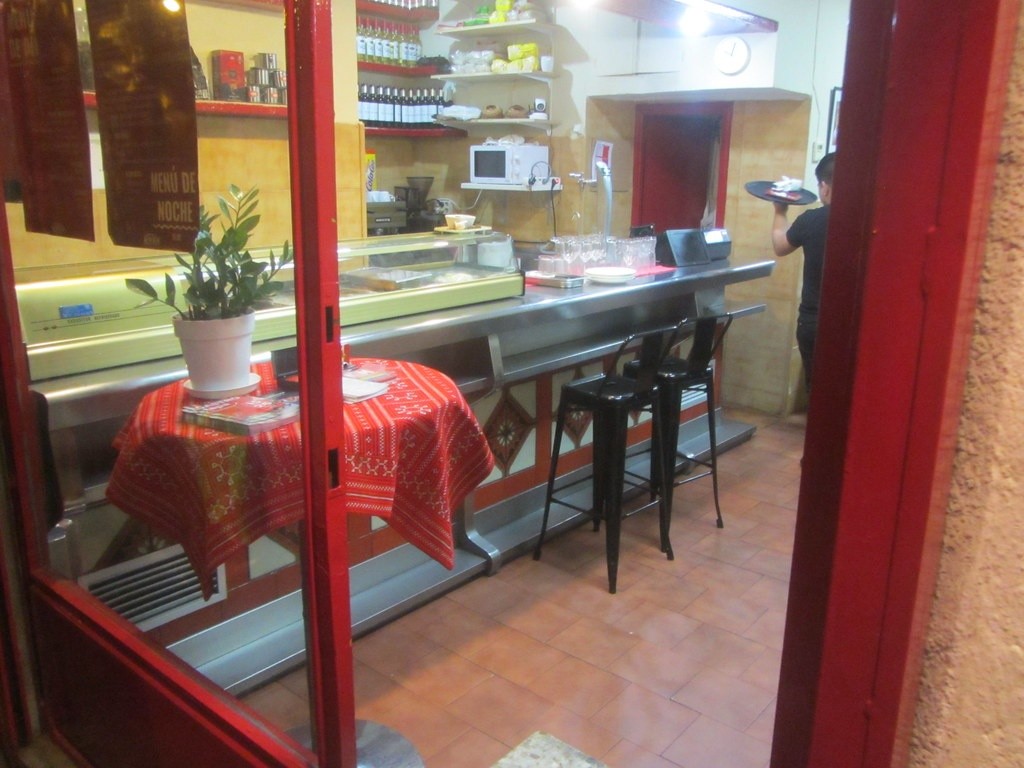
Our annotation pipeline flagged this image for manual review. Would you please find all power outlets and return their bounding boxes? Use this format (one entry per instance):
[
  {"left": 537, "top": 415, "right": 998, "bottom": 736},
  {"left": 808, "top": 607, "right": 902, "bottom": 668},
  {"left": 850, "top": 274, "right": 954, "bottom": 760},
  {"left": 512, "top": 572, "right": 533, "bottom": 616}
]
[{"left": 435, "top": 199, "right": 453, "bottom": 216}]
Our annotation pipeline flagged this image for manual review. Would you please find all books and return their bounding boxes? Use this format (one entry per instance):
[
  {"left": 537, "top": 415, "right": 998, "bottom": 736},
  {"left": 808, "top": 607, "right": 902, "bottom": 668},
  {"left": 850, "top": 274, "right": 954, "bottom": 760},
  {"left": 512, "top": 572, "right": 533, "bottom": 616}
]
[{"left": 183, "top": 393, "right": 300, "bottom": 435}]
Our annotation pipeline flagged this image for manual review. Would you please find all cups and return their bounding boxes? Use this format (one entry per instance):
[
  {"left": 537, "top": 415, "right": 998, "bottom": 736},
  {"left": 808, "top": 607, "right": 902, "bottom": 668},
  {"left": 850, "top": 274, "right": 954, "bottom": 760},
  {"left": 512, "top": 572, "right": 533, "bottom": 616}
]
[
  {"left": 445, "top": 214, "right": 476, "bottom": 229},
  {"left": 539, "top": 234, "right": 658, "bottom": 278},
  {"left": 368, "top": 191, "right": 379, "bottom": 202},
  {"left": 246, "top": 53, "right": 288, "bottom": 105},
  {"left": 379, "top": 191, "right": 395, "bottom": 202}
]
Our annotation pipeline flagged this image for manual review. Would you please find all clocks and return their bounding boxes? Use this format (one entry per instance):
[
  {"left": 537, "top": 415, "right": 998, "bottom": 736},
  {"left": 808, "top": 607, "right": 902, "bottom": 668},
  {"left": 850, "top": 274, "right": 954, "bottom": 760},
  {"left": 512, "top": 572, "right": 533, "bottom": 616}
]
[
  {"left": 713, "top": 36, "right": 751, "bottom": 75},
  {"left": 825, "top": 86, "right": 844, "bottom": 153}
]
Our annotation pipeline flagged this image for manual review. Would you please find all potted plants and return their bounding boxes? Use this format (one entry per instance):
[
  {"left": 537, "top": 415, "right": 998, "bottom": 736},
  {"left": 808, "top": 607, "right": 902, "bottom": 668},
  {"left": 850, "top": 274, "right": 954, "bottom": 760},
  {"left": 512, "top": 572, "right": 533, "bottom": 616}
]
[{"left": 124, "top": 183, "right": 294, "bottom": 397}]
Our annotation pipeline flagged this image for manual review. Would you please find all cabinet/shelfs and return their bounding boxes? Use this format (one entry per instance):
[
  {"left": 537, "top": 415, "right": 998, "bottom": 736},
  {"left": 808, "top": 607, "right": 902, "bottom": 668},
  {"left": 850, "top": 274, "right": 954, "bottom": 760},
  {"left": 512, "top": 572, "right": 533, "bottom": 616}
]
[
  {"left": 357, "top": 0, "right": 467, "bottom": 137},
  {"left": 432, "top": 18, "right": 558, "bottom": 135}
]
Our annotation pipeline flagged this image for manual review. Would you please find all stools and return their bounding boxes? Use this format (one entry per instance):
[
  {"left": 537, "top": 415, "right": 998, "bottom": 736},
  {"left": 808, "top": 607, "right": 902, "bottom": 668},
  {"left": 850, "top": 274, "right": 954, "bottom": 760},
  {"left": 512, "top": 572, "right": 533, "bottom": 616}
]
[
  {"left": 532, "top": 325, "right": 680, "bottom": 594},
  {"left": 622, "top": 313, "right": 735, "bottom": 558}
]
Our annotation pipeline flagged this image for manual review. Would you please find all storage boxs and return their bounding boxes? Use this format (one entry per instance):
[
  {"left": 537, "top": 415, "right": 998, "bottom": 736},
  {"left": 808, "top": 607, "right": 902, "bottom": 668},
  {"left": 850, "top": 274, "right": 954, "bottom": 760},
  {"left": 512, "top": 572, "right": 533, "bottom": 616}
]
[{"left": 212, "top": 50, "right": 246, "bottom": 101}]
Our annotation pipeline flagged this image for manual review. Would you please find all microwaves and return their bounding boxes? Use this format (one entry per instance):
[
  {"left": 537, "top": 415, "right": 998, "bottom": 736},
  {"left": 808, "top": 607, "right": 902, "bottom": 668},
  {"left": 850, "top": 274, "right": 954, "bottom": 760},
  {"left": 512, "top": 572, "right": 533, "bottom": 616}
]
[{"left": 470, "top": 145, "right": 549, "bottom": 184}]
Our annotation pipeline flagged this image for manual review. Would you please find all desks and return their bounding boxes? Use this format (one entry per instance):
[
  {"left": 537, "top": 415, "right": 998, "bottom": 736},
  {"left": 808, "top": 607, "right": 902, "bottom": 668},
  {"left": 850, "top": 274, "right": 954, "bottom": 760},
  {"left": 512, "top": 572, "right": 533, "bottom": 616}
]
[{"left": 104, "top": 358, "right": 493, "bottom": 768}]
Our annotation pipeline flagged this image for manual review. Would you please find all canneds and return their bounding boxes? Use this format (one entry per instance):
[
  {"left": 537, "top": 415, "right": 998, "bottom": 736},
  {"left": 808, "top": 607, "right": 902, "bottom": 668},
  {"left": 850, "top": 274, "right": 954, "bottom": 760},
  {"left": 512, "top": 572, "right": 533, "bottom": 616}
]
[{"left": 248, "top": 53, "right": 287, "bottom": 105}]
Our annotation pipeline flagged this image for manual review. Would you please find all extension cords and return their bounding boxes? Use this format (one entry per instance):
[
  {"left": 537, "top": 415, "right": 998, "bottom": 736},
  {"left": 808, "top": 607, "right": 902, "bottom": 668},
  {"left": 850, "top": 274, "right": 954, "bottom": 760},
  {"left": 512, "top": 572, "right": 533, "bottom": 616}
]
[{"left": 525, "top": 177, "right": 561, "bottom": 186}]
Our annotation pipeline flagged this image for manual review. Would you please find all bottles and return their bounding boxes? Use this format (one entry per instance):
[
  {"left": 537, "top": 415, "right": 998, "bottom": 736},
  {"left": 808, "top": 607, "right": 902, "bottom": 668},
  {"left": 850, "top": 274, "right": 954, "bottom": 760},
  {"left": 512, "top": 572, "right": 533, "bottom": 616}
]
[
  {"left": 358, "top": 84, "right": 453, "bottom": 128},
  {"left": 356, "top": 15, "right": 422, "bottom": 68},
  {"left": 364, "top": 0, "right": 438, "bottom": 11}
]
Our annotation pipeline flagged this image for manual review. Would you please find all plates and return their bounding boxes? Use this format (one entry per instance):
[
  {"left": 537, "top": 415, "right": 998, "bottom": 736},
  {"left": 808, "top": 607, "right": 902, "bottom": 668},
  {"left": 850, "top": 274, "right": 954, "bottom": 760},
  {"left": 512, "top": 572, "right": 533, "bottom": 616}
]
[
  {"left": 743, "top": 180, "right": 818, "bottom": 205},
  {"left": 434, "top": 226, "right": 492, "bottom": 233},
  {"left": 584, "top": 267, "right": 635, "bottom": 283}
]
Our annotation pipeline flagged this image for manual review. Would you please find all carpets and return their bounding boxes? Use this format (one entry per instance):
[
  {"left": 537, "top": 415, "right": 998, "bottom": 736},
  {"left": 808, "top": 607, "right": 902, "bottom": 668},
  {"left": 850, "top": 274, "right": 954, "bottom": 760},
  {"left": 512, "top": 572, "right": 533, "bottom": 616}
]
[{"left": 493, "top": 728, "right": 606, "bottom": 768}]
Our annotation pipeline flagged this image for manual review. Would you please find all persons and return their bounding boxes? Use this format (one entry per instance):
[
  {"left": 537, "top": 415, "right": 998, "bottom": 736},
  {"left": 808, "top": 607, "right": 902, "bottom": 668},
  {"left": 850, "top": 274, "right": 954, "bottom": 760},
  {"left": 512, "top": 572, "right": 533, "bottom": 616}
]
[{"left": 772, "top": 153, "right": 837, "bottom": 468}]
